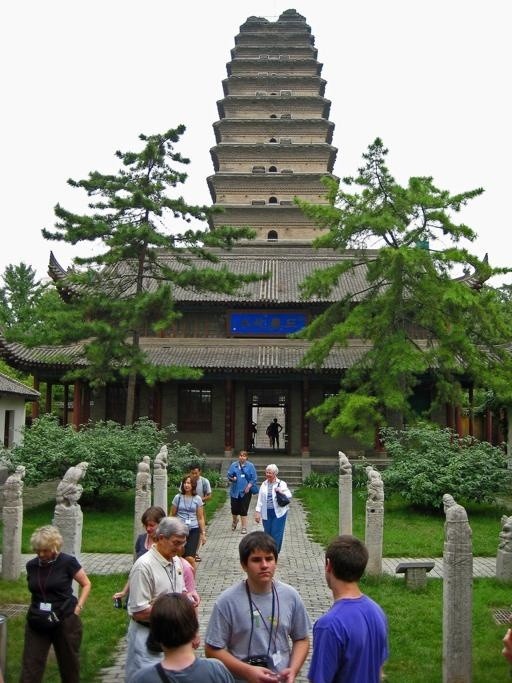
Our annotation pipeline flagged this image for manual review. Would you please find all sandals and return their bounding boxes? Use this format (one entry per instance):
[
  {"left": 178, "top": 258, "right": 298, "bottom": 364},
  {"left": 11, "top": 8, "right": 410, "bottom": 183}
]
[
  {"left": 194, "top": 554, "right": 200, "bottom": 562},
  {"left": 232, "top": 519, "right": 239, "bottom": 532},
  {"left": 241, "top": 528, "right": 247, "bottom": 534}
]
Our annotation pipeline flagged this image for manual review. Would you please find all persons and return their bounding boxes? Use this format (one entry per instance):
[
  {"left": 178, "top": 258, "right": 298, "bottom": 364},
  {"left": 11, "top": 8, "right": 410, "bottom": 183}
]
[
  {"left": 270, "top": 418, "right": 283, "bottom": 449},
  {"left": 266, "top": 422, "right": 273, "bottom": 447},
  {"left": 308, "top": 533, "right": 390, "bottom": 683},
  {"left": 187, "top": 462, "right": 213, "bottom": 505},
  {"left": 172, "top": 476, "right": 207, "bottom": 568},
  {"left": 123, "top": 517, "right": 202, "bottom": 682},
  {"left": 172, "top": 551, "right": 197, "bottom": 611},
  {"left": 111, "top": 506, "right": 167, "bottom": 610},
  {"left": 252, "top": 421, "right": 257, "bottom": 448},
  {"left": 206, "top": 531, "right": 311, "bottom": 683},
  {"left": 124, "top": 591, "right": 234, "bottom": 683},
  {"left": 255, "top": 464, "right": 292, "bottom": 556},
  {"left": 227, "top": 448, "right": 258, "bottom": 535},
  {"left": 19, "top": 523, "right": 91, "bottom": 683}
]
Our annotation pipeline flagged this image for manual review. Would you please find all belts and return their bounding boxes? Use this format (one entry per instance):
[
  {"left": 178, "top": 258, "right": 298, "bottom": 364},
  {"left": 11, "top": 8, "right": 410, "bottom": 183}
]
[{"left": 131, "top": 616, "right": 149, "bottom": 629}]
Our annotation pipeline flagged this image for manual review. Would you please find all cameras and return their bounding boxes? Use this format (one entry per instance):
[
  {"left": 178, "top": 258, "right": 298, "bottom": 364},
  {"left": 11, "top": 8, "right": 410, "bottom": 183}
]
[{"left": 113, "top": 599, "right": 122, "bottom": 608}]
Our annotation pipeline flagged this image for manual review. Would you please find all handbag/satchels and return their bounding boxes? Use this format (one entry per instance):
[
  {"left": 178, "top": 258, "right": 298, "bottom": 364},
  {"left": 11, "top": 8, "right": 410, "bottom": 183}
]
[
  {"left": 276, "top": 491, "right": 291, "bottom": 506},
  {"left": 250, "top": 486, "right": 259, "bottom": 495},
  {"left": 25, "top": 609, "right": 65, "bottom": 635}
]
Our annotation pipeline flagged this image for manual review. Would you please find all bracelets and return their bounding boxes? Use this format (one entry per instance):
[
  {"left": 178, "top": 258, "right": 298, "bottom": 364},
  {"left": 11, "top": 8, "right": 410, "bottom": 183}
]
[
  {"left": 200, "top": 531, "right": 206, "bottom": 534},
  {"left": 77, "top": 603, "right": 82, "bottom": 609}
]
